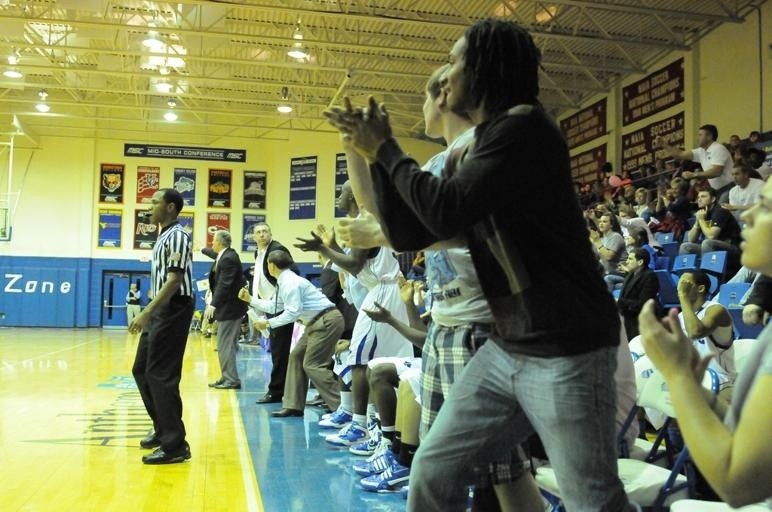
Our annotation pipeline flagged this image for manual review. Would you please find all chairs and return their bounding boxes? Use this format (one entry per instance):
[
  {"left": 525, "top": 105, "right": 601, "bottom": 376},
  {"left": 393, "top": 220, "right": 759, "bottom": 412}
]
[
  {"left": 644, "top": 231, "right": 764, "bottom": 340},
  {"left": 535, "top": 367, "right": 719, "bottom": 510}
]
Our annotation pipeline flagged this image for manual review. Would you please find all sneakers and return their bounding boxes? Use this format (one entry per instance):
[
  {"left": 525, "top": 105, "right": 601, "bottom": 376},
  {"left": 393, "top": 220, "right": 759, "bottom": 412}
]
[
  {"left": 208, "top": 380, "right": 223, "bottom": 387},
  {"left": 142, "top": 442, "right": 191, "bottom": 464},
  {"left": 316, "top": 405, "right": 408, "bottom": 501},
  {"left": 214, "top": 381, "right": 241, "bottom": 390},
  {"left": 202, "top": 330, "right": 212, "bottom": 339},
  {"left": 239, "top": 335, "right": 261, "bottom": 346},
  {"left": 139, "top": 432, "right": 161, "bottom": 450}
]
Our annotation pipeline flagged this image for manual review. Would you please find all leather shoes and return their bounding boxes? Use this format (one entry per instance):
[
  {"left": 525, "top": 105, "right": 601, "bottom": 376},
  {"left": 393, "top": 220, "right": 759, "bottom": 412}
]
[
  {"left": 271, "top": 408, "right": 304, "bottom": 418},
  {"left": 255, "top": 392, "right": 282, "bottom": 404},
  {"left": 306, "top": 398, "right": 325, "bottom": 407}
]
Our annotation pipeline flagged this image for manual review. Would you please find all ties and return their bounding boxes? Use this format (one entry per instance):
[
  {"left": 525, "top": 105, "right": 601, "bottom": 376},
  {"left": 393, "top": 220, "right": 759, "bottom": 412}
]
[{"left": 252, "top": 252, "right": 262, "bottom": 300}]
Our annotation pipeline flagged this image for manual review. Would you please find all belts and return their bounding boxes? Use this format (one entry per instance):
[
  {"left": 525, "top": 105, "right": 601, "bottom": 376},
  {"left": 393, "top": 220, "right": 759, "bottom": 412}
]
[{"left": 307, "top": 305, "right": 337, "bottom": 324}]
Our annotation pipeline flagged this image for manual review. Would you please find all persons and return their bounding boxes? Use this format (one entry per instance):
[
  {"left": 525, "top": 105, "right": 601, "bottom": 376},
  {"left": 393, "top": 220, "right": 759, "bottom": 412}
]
[
  {"left": 200, "top": 265, "right": 272, "bottom": 352},
  {"left": 128, "top": 188, "right": 194, "bottom": 465},
  {"left": 236, "top": 249, "right": 345, "bottom": 417},
  {"left": 252, "top": 222, "right": 300, "bottom": 404},
  {"left": 192, "top": 230, "right": 248, "bottom": 390},
  {"left": 125, "top": 283, "right": 142, "bottom": 334},
  {"left": 295, "top": 19, "right": 771, "bottom": 512}
]
